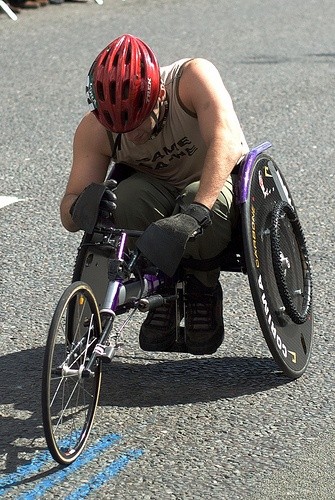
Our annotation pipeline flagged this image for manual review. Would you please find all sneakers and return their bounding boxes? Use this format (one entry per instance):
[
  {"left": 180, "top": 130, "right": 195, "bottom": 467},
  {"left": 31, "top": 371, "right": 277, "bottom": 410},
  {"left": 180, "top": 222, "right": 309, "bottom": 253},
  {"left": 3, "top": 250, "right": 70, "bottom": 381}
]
[{"left": 138, "top": 280, "right": 224, "bottom": 355}]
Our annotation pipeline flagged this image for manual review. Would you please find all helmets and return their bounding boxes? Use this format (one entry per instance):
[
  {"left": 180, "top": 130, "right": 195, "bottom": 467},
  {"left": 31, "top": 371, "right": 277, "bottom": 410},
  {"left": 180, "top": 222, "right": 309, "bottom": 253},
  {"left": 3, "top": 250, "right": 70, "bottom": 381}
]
[{"left": 85, "top": 33, "right": 160, "bottom": 133}]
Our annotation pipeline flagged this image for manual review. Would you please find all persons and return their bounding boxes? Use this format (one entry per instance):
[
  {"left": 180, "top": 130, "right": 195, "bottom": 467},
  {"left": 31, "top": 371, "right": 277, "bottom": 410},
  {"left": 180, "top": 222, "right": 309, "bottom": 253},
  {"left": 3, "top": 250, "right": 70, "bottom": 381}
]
[{"left": 60, "top": 33, "right": 249, "bottom": 356}]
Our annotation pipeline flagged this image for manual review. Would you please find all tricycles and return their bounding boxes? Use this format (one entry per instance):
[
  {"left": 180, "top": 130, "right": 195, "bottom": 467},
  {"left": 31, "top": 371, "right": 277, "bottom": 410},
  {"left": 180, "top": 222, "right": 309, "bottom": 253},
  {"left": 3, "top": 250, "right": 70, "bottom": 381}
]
[{"left": 40, "top": 142, "right": 315, "bottom": 465}]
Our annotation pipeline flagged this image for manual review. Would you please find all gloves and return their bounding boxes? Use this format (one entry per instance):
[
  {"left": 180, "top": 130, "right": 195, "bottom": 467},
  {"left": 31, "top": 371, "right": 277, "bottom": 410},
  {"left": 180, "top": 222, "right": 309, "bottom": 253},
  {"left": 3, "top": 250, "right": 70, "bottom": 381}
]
[
  {"left": 135, "top": 204, "right": 212, "bottom": 278},
  {"left": 69, "top": 178, "right": 117, "bottom": 231}
]
